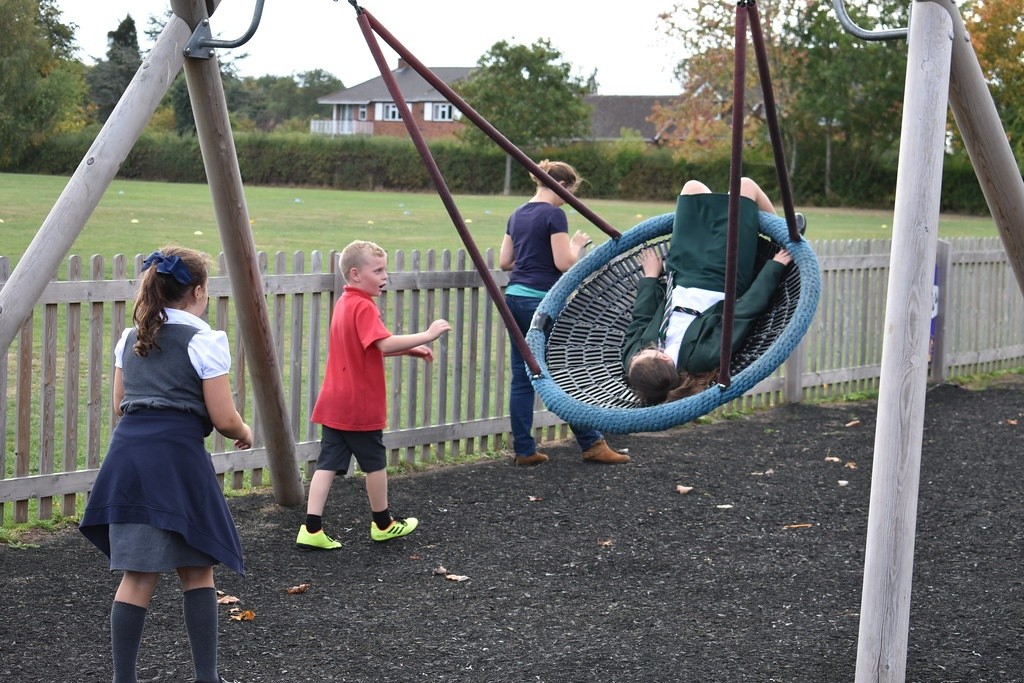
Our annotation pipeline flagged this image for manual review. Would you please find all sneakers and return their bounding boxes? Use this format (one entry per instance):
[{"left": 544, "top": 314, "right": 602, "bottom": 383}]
[
  {"left": 296, "top": 525, "right": 342, "bottom": 549},
  {"left": 371, "top": 515, "right": 418, "bottom": 542}
]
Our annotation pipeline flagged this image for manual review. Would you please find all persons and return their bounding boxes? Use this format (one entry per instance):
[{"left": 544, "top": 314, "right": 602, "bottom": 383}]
[
  {"left": 499, "top": 159, "right": 630, "bottom": 467},
  {"left": 624, "top": 176, "right": 807, "bottom": 404},
  {"left": 295, "top": 241, "right": 451, "bottom": 550},
  {"left": 84, "top": 247, "right": 251, "bottom": 683}
]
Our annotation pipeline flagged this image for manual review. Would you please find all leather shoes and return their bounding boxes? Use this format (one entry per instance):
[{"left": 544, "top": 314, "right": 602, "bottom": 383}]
[{"left": 795, "top": 213, "right": 806, "bottom": 236}]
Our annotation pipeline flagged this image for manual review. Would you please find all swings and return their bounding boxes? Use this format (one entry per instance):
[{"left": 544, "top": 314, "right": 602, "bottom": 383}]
[{"left": 350, "top": 0, "right": 819, "bottom": 432}]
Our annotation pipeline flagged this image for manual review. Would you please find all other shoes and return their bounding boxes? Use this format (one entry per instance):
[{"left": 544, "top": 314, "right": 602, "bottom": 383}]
[
  {"left": 583, "top": 439, "right": 630, "bottom": 463},
  {"left": 516, "top": 451, "right": 548, "bottom": 466}
]
